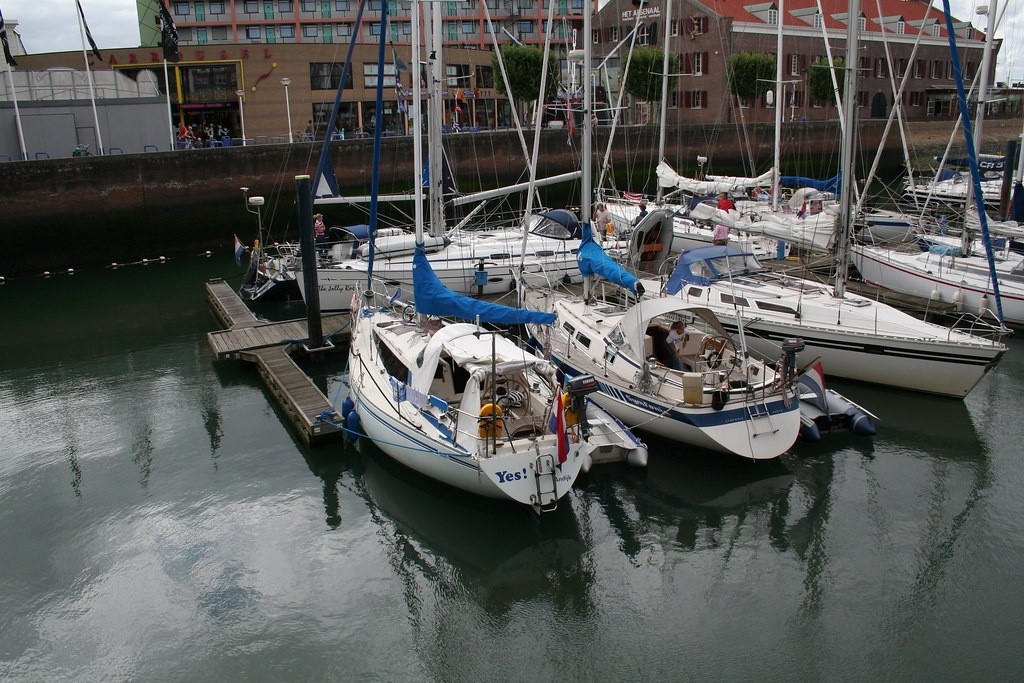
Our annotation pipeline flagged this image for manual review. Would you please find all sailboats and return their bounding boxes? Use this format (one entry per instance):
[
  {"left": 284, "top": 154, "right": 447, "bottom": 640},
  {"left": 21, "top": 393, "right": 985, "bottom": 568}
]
[{"left": 237, "top": 0, "right": 1024, "bottom": 515}]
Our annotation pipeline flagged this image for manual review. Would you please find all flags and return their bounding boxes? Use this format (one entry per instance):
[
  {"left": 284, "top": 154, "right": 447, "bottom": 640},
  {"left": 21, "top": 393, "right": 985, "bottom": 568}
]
[
  {"left": 157, "top": 0, "right": 182, "bottom": 64},
  {"left": 0, "top": 10, "right": 18, "bottom": 68},
  {"left": 568, "top": 103, "right": 575, "bottom": 140},
  {"left": 397, "top": 95, "right": 409, "bottom": 115},
  {"left": 546, "top": 387, "right": 571, "bottom": 464},
  {"left": 395, "top": 74, "right": 409, "bottom": 97},
  {"left": 393, "top": 50, "right": 409, "bottom": 72},
  {"left": 76, "top": 0, "right": 104, "bottom": 62},
  {"left": 456, "top": 88, "right": 469, "bottom": 105}
]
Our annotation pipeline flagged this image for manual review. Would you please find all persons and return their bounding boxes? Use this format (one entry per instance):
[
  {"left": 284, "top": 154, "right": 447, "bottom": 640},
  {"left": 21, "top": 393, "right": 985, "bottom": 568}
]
[
  {"left": 178, "top": 122, "right": 187, "bottom": 141},
  {"left": 665, "top": 321, "right": 688, "bottom": 371},
  {"left": 185, "top": 126, "right": 196, "bottom": 148},
  {"left": 592, "top": 114, "right": 598, "bottom": 127},
  {"left": 716, "top": 191, "right": 737, "bottom": 213},
  {"left": 632, "top": 205, "right": 648, "bottom": 227},
  {"left": 592, "top": 204, "right": 611, "bottom": 241},
  {"left": 313, "top": 214, "right": 325, "bottom": 255},
  {"left": 197, "top": 137, "right": 203, "bottom": 148},
  {"left": 307, "top": 119, "right": 315, "bottom": 141}
]
